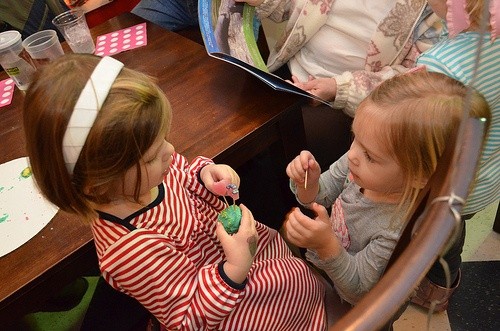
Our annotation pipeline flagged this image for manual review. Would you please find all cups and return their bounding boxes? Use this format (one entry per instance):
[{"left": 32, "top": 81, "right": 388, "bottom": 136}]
[
  {"left": 52, "top": 9, "right": 95, "bottom": 55},
  {"left": 22, "top": 30, "right": 65, "bottom": 66},
  {"left": 0, "top": 31, "right": 37, "bottom": 91}
]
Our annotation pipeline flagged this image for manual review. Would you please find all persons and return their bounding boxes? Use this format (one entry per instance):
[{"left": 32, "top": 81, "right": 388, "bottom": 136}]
[
  {"left": 233, "top": 0, "right": 444, "bottom": 119},
  {"left": 130, "top": 0, "right": 198, "bottom": 37},
  {"left": 412, "top": 0, "right": 500, "bottom": 314},
  {"left": 284, "top": 71, "right": 492, "bottom": 331},
  {"left": 23, "top": 53, "right": 328, "bottom": 331}
]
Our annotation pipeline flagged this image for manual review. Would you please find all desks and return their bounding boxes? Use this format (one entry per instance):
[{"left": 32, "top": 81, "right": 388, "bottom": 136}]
[{"left": 0, "top": 12, "right": 307, "bottom": 331}]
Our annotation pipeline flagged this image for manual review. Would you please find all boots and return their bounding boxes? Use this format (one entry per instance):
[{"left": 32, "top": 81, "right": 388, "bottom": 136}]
[{"left": 411, "top": 269, "right": 461, "bottom": 313}]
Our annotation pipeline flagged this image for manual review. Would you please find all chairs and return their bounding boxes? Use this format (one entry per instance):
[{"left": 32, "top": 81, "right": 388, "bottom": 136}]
[{"left": 328, "top": 117, "right": 484, "bottom": 331}]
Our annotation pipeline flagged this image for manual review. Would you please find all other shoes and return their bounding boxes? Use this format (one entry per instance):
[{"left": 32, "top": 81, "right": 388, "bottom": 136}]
[{"left": 26, "top": 275, "right": 89, "bottom": 312}]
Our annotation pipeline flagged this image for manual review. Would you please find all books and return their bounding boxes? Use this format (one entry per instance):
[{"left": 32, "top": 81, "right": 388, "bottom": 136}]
[{"left": 197, "top": 0, "right": 335, "bottom": 111}]
